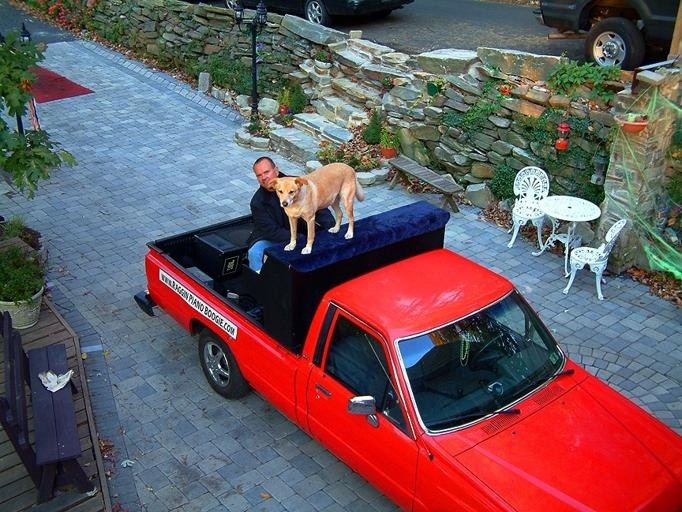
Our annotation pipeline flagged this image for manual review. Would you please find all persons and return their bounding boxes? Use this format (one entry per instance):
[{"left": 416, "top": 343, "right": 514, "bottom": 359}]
[{"left": 244, "top": 157, "right": 336, "bottom": 321}]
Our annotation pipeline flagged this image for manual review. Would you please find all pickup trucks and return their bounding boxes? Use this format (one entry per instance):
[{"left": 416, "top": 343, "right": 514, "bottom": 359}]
[{"left": 135, "top": 209, "right": 682, "bottom": 512}]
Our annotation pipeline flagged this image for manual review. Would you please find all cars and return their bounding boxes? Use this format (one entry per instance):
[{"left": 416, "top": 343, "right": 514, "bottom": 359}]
[
  {"left": 533, "top": 1, "right": 679, "bottom": 68},
  {"left": 225, "top": 0, "right": 416, "bottom": 26}
]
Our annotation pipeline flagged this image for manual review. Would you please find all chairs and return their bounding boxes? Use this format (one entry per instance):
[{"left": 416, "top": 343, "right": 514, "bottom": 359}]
[
  {"left": 506, "top": 166, "right": 550, "bottom": 250},
  {"left": 563, "top": 218, "right": 628, "bottom": 301},
  {"left": 328, "top": 317, "right": 513, "bottom": 422}
]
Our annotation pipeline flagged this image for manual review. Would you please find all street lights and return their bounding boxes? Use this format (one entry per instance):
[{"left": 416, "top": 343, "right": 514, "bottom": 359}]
[
  {"left": 0, "top": 23, "right": 31, "bottom": 139},
  {"left": 233, "top": 0, "right": 268, "bottom": 121}
]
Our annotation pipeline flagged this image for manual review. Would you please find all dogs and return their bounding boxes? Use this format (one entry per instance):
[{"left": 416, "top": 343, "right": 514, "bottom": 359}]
[{"left": 266, "top": 162, "right": 365, "bottom": 255}]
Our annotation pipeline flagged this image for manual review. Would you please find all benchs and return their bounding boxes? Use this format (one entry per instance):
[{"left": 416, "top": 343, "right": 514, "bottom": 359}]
[
  {"left": 388, "top": 152, "right": 467, "bottom": 208},
  {"left": 0, "top": 311, "right": 94, "bottom": 505}
]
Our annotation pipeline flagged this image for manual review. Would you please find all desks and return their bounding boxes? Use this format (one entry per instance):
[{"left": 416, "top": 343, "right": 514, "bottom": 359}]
[{"left": 531, "top": 196, "right": 602, "bottom": 277}]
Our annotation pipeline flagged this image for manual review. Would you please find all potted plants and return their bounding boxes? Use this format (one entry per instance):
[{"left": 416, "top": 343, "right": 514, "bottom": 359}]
[
  {"left": 314, "top": 50, "right": 334, "bottom": 69},
  {"left": 0, "top": 244, "right": 45, "bottom": 329},
  {"left": 380, "top": 132, "right": 400, "bottom": 158}
]
